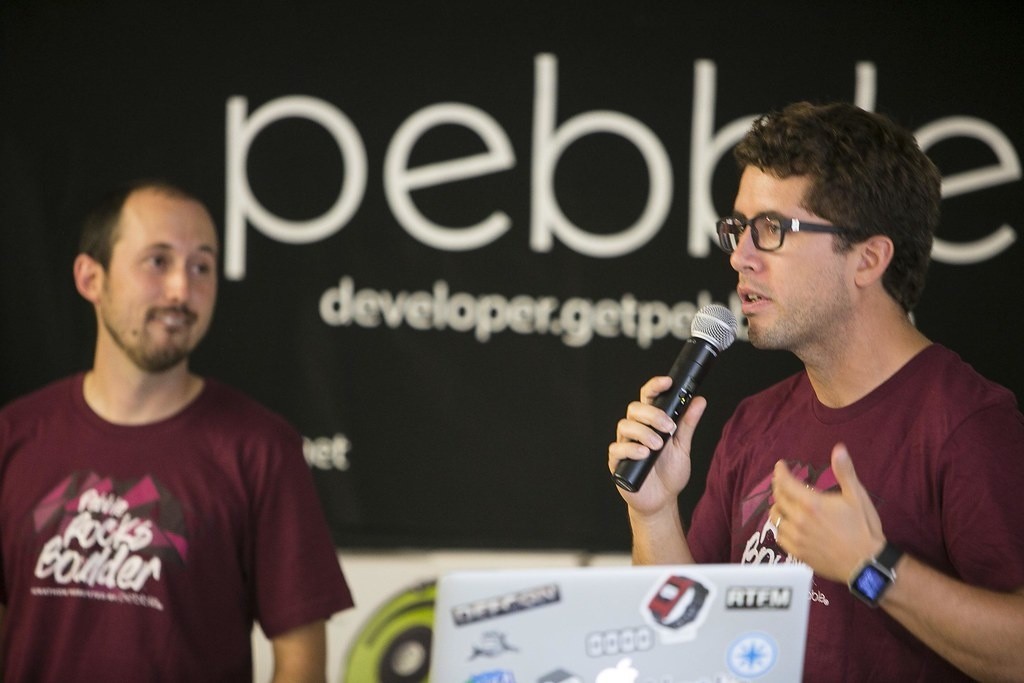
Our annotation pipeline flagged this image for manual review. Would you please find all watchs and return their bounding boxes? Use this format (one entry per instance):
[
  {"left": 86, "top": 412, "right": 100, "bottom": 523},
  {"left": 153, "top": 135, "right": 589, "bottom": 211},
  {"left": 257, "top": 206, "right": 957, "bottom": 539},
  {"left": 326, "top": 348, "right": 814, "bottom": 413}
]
[{"left": 846, "top": 541, "right": 906, "bottom": 609}]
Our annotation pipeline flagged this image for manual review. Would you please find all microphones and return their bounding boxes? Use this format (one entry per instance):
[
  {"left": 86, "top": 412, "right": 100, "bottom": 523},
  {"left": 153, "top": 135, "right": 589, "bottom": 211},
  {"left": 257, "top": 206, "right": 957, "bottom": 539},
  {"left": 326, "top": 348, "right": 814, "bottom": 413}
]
[{"left": 611, "top": 304, "right": 736, "bottom": 493}]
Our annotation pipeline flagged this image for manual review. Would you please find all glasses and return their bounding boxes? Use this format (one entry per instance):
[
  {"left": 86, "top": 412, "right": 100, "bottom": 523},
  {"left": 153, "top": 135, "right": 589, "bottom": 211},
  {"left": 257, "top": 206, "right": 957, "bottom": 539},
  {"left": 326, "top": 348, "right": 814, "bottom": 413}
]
[{"left": 715, "top": 214, "right": 873, "bottom": 254}]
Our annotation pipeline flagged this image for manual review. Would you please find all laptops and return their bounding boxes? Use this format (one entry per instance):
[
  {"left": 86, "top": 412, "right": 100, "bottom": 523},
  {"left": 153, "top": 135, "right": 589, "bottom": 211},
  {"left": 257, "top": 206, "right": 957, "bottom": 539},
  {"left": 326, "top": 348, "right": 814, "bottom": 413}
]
[{"left": 426, "top": 563, "right": 814, "bottom": 683}]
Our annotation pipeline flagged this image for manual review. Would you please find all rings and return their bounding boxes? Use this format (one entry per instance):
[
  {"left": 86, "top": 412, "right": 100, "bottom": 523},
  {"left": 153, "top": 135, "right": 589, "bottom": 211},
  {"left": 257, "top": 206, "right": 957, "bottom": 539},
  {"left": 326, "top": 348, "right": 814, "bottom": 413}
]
[{"left": 775, "top": 517, "right": 783, "bottom": 530}]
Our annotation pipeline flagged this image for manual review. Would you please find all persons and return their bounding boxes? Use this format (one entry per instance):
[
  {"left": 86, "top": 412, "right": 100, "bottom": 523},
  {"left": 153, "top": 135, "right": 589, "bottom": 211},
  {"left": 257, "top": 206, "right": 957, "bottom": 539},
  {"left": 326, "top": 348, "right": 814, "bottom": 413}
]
[
  {"left": 0, "top": 169, "right": 356, "bottom": 683},
  {"left": 606, "top": 102, "right": 1023, "bottom": 683}
]
